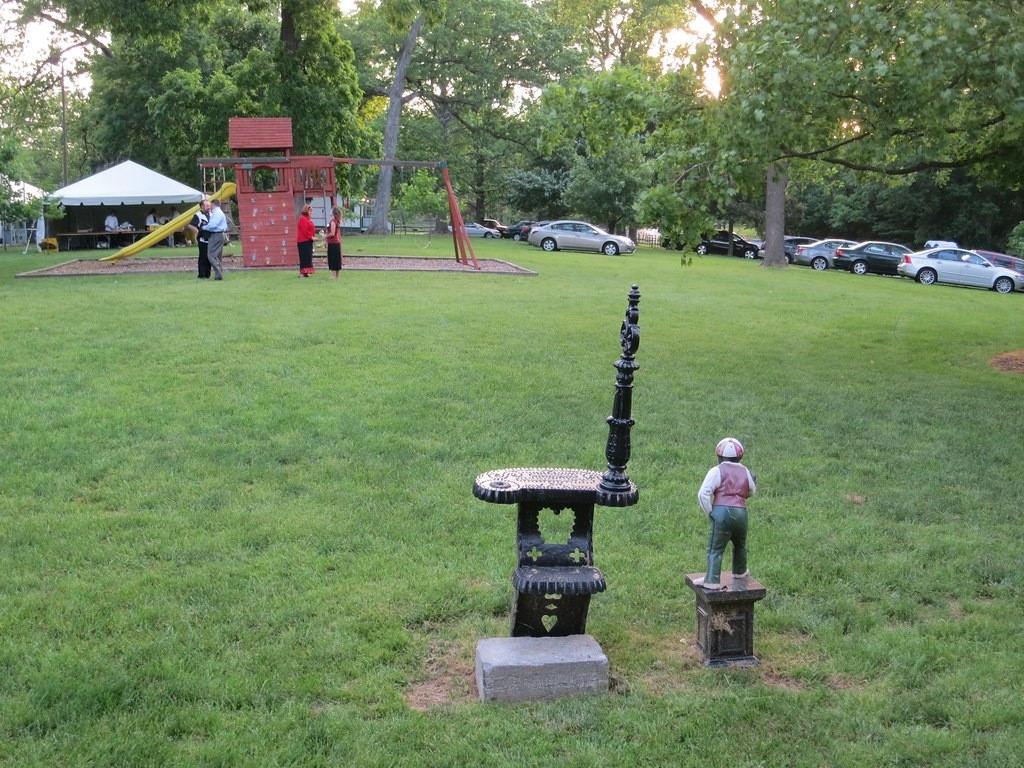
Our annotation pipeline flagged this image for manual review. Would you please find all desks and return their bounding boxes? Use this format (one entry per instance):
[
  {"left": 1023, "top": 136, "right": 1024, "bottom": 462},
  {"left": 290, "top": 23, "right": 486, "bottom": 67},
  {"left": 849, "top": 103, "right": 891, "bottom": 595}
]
[{"left": 56, "top": 230, "right": 184, "bottom": 251}]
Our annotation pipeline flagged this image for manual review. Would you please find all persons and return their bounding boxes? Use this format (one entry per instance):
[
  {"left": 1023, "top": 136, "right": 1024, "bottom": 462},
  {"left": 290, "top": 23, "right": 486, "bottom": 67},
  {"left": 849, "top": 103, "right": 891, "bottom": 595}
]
[
  {"left": 146, "top": 207, "right": 188, "bottom": 247},
  {"left": 297, "top": 205, "right": 319, "bottom": 277},
  {"left": 105, "top": 210, "right": 118, "bottom": 249},
  {"left": 189, "top": 198, "right": 227, "bottom": 280},
  {"left": 322, "top": 206, "right": 343, "bottom": 280},
  {"left": 692, "top": 438, "right": 756, "bottom": 589}
]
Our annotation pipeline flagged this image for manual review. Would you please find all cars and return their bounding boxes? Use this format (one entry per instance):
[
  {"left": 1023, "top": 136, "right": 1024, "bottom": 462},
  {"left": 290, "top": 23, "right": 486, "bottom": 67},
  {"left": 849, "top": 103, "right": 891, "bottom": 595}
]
[
  {"left": 683, "top": 229, "right": 759, "bottom": 259},
  {"left": 923, "top": 240, "right": 1024, "bottom": 274},
  {"left": 526, "top": 219, "right": 636, "bottom": 257},
  {"left": 831, "top": 239, "right": 916, "bottom": 277},
  {"left": 896, "top": 247, "right": 1024, "bottom": 294},
  {"left": 662, "top": 232, "right": 692, "bottom": 251},
  {"left": 447, "top": 216, "right": 595, "bottom": 242},
  {"left": 759, "top": 234, "right": 833, "bottom": 266},
  {"left": 793, "top": 239, "right": 895, "bottom": 272}
]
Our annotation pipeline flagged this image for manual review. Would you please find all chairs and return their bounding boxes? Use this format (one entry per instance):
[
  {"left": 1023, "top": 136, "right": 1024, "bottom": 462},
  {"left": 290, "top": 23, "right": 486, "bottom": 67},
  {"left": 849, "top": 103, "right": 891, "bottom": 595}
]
[
  {"left": 575, "top": 226, "right": 582, "bottom": 232},
  {"left": 882, "top": 249, "right": 889, "bottom": 255}
]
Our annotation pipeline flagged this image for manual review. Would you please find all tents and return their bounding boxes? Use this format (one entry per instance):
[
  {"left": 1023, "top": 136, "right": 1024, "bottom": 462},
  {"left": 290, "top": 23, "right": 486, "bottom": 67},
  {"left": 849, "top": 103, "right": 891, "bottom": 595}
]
[
  {"left": 37, "top": 160, "right": 211, "bottom": 252},
  {"left": 0, "top": 173, "right": 50, "bottom": 243}
]
